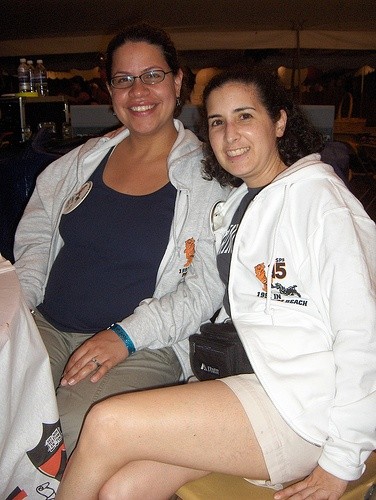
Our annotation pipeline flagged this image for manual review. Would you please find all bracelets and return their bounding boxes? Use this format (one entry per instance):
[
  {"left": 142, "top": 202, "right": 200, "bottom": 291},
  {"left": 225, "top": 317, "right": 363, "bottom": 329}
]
[{"left": 110, "top": 324, "right": 135, "bottom": 356}]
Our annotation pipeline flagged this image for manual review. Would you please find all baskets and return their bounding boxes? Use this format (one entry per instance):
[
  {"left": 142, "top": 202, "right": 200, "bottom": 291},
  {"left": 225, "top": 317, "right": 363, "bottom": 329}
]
[{"left": 334, "top": 93, "right": 366, "bottom": 140}]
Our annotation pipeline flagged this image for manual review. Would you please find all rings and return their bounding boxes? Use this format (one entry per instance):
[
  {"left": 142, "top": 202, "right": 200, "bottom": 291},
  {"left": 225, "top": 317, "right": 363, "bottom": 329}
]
[{"left": 91, "top": 360, "right": 100, "bottom": 367}]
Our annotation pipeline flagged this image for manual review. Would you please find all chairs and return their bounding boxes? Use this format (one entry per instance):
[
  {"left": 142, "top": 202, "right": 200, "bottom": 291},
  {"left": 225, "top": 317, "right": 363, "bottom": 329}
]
[
  {"left": 335, "top": 139, "right": 376, "bottom": 211},
  {"left": 173, "top": 449, "right": 376, "bottom": 500}
]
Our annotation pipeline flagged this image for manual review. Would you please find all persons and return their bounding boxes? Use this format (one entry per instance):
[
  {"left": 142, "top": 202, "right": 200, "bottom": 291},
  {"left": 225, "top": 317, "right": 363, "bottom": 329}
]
[
  {"left": 55, "top": 60, "right": 376, "bottom": 500},
  {"left": 14, "top": 24, "right": 233, "bottom": 461},
  {"left": 299, "top": 67, "right": 325, "bottom": 106},
  {"left": 66, "top": 76, "right": 111, "bottom": 105},
  {"left": 180, "top": 60, "right": 226, "bottom": 105}
]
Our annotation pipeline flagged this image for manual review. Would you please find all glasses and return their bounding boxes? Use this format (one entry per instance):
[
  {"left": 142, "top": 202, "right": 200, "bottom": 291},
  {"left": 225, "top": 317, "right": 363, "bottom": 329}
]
[{"left": 110, "top": 69, "right": 173, "bottom": 89}]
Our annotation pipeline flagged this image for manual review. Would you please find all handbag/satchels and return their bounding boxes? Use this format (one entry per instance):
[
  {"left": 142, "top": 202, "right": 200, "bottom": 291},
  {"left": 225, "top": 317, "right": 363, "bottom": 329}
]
[{"left": 187, "top": 322, "right": 255, "bottom": 383}]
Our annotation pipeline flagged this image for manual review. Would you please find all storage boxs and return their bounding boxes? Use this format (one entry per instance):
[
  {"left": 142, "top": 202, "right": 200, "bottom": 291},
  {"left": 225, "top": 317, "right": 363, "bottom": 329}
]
[{"left": 0, "top": 95, "right": 70, "bottom": 146}]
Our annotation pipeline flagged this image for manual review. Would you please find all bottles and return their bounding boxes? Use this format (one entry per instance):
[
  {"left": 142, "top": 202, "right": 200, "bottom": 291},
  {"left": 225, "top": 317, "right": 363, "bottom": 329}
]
[{"left": 17, "top": 58, "right": 49, "bottom": 96}]
